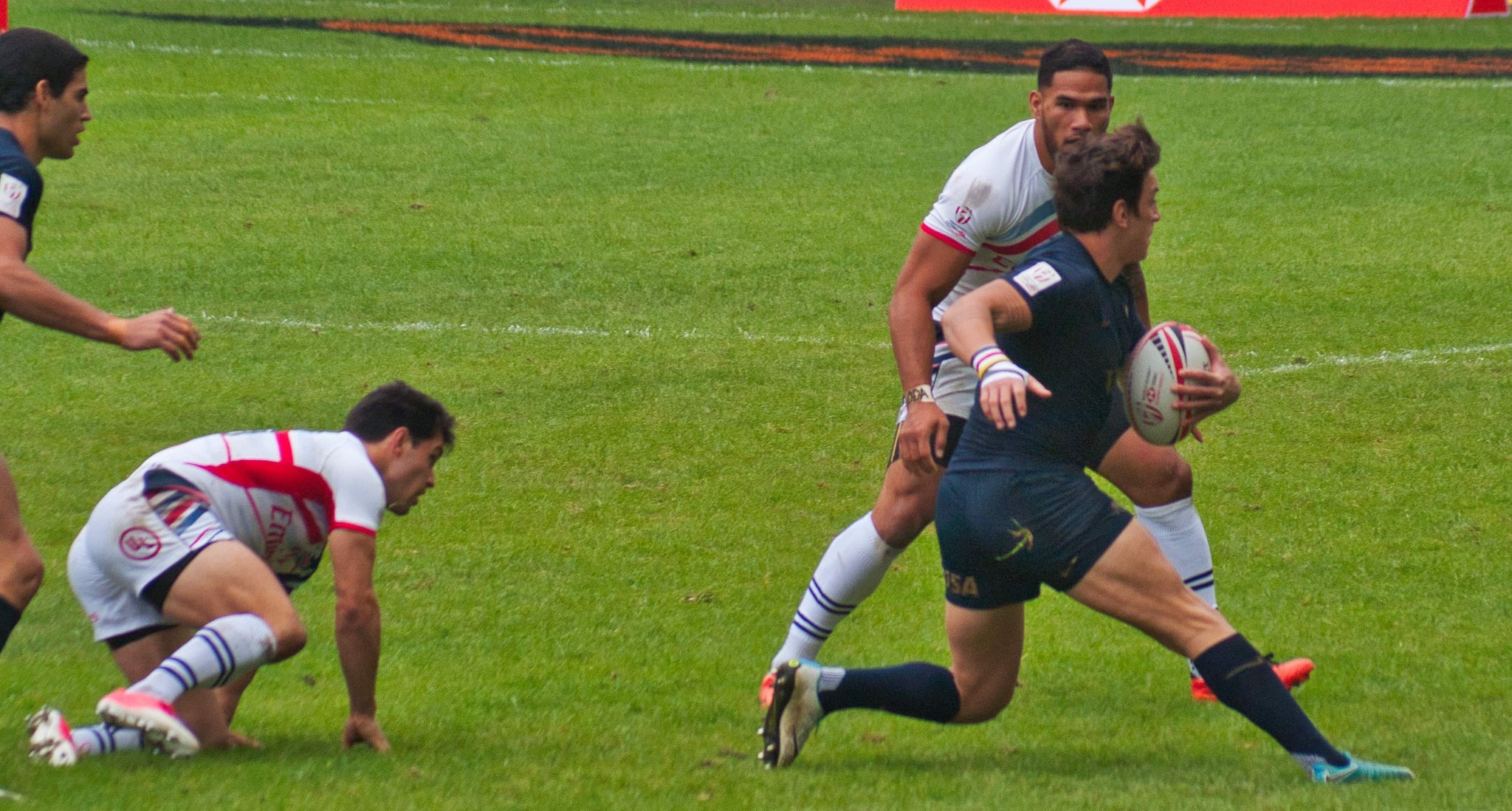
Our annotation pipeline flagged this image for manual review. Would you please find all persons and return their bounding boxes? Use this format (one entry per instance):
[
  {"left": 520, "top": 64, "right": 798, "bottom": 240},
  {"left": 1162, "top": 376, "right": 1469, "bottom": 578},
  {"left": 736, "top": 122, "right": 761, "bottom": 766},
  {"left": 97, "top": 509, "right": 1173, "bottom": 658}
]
[
  {"left": 24, "top": 378, "right": 464, "bottom": 767},
  {"left": 0, "top": 26, "right": 202, "bottom": 651},
  {"left": 754, "top": 37, "right": 1422, "bottom": 785}
]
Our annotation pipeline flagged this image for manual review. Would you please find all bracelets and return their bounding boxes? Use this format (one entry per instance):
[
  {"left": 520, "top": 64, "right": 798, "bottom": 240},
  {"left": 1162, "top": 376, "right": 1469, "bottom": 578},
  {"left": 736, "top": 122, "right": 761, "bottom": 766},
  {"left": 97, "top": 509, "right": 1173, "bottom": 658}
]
[
  {"left": 978, "top": 357, "right": 1011, "bottom": 380},
  {"left": 905, "top": 384, "right": 935, "bottom": 407}
]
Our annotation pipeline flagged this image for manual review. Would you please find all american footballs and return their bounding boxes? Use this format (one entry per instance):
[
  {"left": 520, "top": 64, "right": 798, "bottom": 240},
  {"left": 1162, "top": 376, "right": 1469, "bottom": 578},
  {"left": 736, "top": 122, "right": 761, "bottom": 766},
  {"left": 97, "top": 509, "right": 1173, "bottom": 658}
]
[{"left": 1120, "top": 318, "right": 1210, "bottom": 446}]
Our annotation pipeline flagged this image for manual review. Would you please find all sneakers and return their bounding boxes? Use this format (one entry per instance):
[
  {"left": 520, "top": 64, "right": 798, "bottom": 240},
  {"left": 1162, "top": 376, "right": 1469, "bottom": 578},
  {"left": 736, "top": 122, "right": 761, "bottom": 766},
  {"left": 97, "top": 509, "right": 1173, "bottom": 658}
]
[
  {"left": 1191, "top": 653, "right": 1315, "bottom": 701},
  {"left": 23, "top": 704, "right": 91, "bottom": 766},
  {"left": 758, "top": 659, "right": 824, "bottom": 771},
  {"left": 760, "top": 671, "right": 777, "bottom": 710},
  {"left": 94, "top": 687, "right": 201, "bottom": 760},
  {"left": 1294, "top": 745, "right": 1415, "bottom": 786}
]
